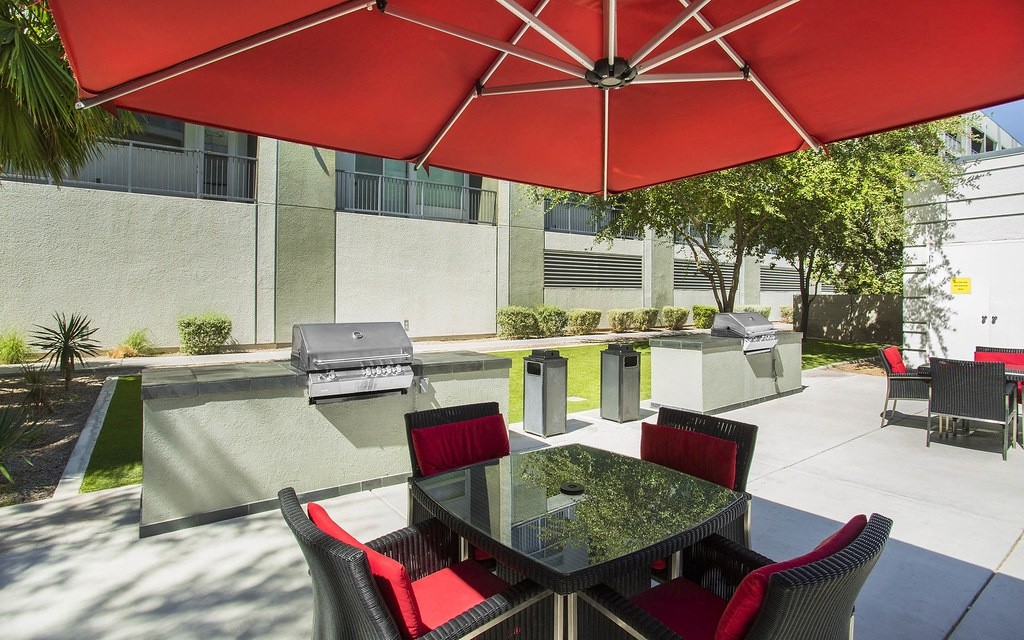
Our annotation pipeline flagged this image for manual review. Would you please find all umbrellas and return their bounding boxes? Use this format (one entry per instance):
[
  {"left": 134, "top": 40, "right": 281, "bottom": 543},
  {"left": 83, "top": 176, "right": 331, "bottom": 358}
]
[{"left": 49, "top": 0, "right": 1024, "bottom": 200}]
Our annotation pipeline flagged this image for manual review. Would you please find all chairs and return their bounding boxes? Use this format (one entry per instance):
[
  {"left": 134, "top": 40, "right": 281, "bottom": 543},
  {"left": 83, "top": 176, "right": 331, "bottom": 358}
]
[
  {"left": 404, "top": 402, "right": 512, "bottom": 569},
  {"left": 641, "top": 407, "right": 759, "bottom": 584},
  {"left": 278, "top": 486, "right": 564, "bottom": 640},
  {"left": 567, "top": 512, "right": 893, "bottom": 640},
  {"left": 878, "top": 345, "right": 1024, "bottom": 461}
]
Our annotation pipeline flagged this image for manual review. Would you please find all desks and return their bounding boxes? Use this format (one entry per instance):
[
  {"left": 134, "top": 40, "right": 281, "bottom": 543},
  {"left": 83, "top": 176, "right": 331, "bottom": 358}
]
[
  {"left": 409, "top": 444, "right": 750, "bottom": 640},
  {"left": 918, "top": 365, "right": 1024, "bottom": 443}
]
